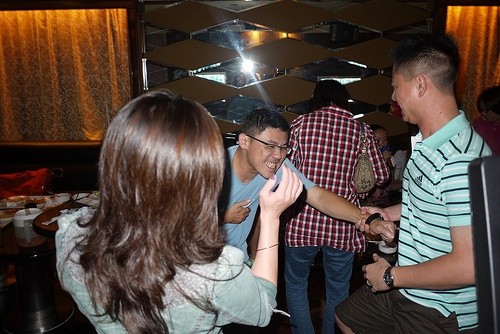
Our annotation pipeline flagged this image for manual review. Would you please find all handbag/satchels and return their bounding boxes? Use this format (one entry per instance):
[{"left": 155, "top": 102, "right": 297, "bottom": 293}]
[{"left": 353, "top": 120, "right": 375, "bottom": 193}]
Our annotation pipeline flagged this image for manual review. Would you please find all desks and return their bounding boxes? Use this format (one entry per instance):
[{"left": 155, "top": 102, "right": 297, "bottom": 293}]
[{"left": 0, "top": 195, "right": 90, "bottom": 334}]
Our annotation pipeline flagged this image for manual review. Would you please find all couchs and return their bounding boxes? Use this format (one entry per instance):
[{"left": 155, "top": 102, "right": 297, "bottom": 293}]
[{"left": 0, "top": 140, "right": 102, "bottom": 194}]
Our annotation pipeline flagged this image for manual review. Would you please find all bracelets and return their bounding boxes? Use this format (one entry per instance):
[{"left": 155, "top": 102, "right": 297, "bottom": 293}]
[{"left": 256, "top": 242, "right": 280, "bottom": 252}]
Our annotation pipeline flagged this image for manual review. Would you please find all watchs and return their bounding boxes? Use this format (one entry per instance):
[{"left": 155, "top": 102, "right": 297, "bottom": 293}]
[
  {"left": 364, "top": 212, "right": 384, "bottom": 226},
  {"left": 383, "top": 265, "right": 396, "bottom": 291}
]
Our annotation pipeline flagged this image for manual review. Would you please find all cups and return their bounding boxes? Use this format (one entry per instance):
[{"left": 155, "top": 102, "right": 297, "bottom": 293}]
[
  {"left": 378, "top": 241, "right": 397, "bottom": 267},
  {"left": 54, "top": 193, "right": 70, "bottom": 203},
  {"left": 13, "top": 208, "right": 45, "bottom": 247}
]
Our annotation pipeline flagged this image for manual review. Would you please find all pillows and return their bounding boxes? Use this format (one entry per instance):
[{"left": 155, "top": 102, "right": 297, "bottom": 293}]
[{"left": 0, "top": 168, "right": 51, "bottom": 201}]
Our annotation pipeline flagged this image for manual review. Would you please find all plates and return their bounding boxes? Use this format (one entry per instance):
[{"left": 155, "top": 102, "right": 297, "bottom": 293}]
[{"left": 0, "top": 218, "right": 13, "bottom": 228}]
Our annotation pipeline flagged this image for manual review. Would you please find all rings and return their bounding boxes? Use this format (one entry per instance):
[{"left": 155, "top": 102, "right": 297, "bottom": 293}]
[{"left": 367, "top": 280, "right": 372, "bottom": 287}]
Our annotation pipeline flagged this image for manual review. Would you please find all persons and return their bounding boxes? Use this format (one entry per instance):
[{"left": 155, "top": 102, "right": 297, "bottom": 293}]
[
  {"left": 54, "top": 91, "right": 304, "bottom": 334},
  {"left": 472, "top": 86, "right": 500, "bottom": 157},
  {"left": 359, "top": 124, "right": 410, "bottom": 218},
  {"left": 218, "top": 108, "right": 396, "bottom": 333},
  {"left": 333, "top": 32, "right": 494, "bottom": 334},
  {"left": 284, "top": 79, "right": 391, "bottom": 334}
]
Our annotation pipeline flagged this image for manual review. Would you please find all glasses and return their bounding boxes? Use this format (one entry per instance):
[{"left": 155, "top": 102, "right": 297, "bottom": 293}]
[
  {"left": 246, "top": 135, "right": 292, "bottom": 154},
  {"left": 478, "top": 107, "right": 493, "bottom": 114}
]
